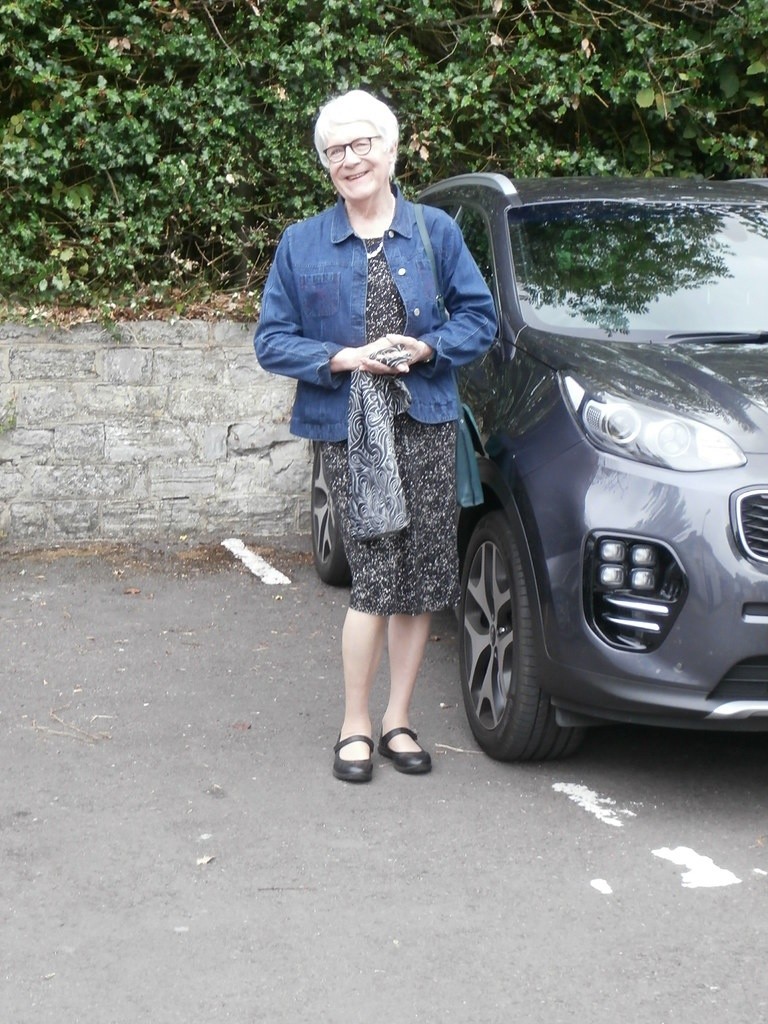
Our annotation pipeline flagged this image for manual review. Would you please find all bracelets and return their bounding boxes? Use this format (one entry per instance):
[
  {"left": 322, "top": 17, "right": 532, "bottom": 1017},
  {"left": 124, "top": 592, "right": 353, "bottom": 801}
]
[{"left": 422, "top": 351, "right": 435, "bottom": 363}]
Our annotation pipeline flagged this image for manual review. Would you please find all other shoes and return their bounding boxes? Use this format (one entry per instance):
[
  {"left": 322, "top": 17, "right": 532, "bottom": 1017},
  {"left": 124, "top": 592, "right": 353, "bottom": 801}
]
[
  {"left": 332, "top": 731, "right": 374, "bottom": 781},
  {"left": 378, "top": 725, "right": 431, "bottom": 773}
]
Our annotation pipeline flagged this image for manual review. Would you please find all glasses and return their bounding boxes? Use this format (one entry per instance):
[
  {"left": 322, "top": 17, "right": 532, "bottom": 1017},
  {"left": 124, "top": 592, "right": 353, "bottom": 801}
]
[{"left": 323, "top": 136, "right": 380, "bottom": 163}]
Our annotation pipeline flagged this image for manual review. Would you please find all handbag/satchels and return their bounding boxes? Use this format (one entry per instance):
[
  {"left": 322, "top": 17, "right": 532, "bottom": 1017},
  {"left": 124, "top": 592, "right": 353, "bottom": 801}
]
[{"left": 457, "top": 403, "right": 486, "bottom": 508}]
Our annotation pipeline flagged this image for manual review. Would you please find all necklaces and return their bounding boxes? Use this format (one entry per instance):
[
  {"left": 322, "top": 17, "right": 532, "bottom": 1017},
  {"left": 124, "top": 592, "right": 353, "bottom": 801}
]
[{"left": 367, "top": 193, "right": 396, "bottom": 260}]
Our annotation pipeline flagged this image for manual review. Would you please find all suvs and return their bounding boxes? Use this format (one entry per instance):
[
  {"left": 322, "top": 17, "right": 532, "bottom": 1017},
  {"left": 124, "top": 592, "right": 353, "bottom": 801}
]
[{"left": 301, "top": 172, "right": 767, "bottom": 760}]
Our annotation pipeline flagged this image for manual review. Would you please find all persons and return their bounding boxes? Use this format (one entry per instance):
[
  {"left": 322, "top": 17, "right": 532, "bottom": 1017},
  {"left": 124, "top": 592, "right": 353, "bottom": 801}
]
[{"left": 253, "top": 90, "right": 499, "bottom": 782}]
